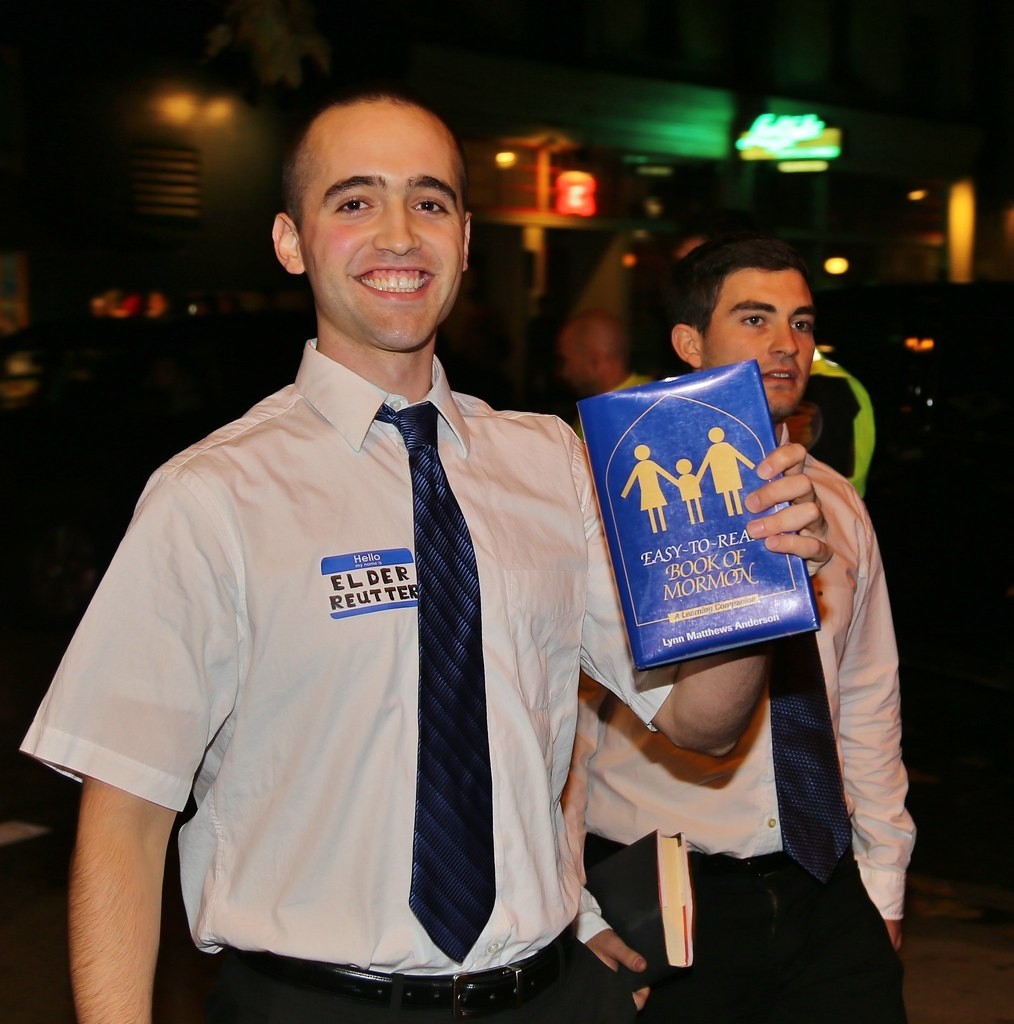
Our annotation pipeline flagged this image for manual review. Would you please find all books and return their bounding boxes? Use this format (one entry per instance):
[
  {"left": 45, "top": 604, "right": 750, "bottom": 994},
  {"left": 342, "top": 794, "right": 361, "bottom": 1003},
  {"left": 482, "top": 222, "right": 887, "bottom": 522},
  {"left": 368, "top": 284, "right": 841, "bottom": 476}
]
[{"left": 575, "top": 358, "right": 822, "bottom": 674}]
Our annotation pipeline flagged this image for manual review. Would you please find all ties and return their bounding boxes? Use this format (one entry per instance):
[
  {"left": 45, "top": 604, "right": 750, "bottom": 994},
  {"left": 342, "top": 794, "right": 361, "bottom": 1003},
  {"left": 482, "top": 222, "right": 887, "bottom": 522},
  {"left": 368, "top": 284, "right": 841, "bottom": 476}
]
[
  {"left": 763, "top": 631, "right": 852, "bottom": 886},
  {"left": 370, "top": 402, "right": 497, "bottom": 964}
]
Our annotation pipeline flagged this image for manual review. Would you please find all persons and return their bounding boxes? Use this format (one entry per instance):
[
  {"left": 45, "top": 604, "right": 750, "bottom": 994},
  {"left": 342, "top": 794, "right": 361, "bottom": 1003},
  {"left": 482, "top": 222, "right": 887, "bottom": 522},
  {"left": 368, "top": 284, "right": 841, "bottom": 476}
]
[{"left": 13, "top": 89, "right": 925, "bottom": 1023}]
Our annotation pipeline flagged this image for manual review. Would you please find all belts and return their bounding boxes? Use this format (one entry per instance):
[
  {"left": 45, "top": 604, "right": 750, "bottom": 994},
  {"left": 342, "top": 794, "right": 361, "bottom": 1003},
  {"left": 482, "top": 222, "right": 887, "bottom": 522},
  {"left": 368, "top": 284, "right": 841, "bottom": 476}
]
[
  {"left": 692, "top": 852, "right": 799, "bottom": 879},
  {"left": 235, "top": 925, "right": 573, "bottom": 1020}
]
